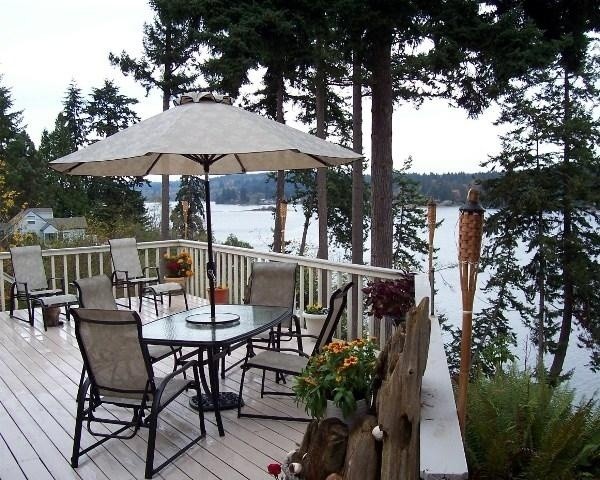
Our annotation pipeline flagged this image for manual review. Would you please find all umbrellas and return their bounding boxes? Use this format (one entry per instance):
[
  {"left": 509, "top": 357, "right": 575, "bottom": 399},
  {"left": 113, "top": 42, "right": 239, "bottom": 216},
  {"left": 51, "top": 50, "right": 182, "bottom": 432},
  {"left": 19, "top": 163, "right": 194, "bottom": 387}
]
[{"left": 47, "top": 91, "right": 368, "bottom": 318}]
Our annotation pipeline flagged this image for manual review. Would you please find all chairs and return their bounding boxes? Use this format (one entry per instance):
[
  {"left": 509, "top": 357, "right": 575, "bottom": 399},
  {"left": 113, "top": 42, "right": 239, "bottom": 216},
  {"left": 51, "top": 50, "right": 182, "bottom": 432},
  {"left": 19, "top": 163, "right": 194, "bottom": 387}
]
[
  {"left": 70, "top": 306, "right": 207, "bottom": 480},
  {"left": 218, "top": 262, "right": 305, "bottom": 385},
  {"left": 9, "top": 246, "right": 79, "bottom": 331},
  {"left": 236, "top": 282, "right": 355, "bottom": 421},
  {"left": 75, "top": 277, "right": 190, "bottom": 402},
  {"left": 106, "top": 238, "right": 188, "bottom": 315}
]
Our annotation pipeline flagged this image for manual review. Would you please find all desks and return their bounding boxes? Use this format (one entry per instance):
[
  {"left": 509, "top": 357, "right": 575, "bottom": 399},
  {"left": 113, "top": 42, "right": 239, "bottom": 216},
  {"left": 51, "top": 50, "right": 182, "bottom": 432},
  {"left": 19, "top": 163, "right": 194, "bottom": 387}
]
[{"left": 135, "top": 304, "right": 303, "bottom": 436}]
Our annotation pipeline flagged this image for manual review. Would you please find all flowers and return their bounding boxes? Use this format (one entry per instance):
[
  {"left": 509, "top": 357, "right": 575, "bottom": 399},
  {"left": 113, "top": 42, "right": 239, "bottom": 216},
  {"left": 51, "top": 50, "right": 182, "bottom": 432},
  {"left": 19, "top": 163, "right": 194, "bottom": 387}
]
[
  {"left": 360, "top": 265, "right": 419, "bottom": 328},
  {"left": 161, "top": 251, "right": 194, "bottom": 277},
  {"left": 301, "top": 303, "right": 332, "bottom": 313},
  {"left": 290, "top": 332, "right": 380, "bottom": 416}
]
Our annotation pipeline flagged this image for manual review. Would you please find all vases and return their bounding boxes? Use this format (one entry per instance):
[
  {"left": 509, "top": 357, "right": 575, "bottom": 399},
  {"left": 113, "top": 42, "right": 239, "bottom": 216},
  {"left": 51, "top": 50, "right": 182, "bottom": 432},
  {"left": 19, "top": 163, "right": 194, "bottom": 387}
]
[
  {"left": 324, "top": 398, "right": 368, "bottom": 434},
  {"left": 302, "top": 312, "right": 329, "bottom": 336},
  {"left": 163, "top": 276, "right": 185, "bottom": 291}
]
